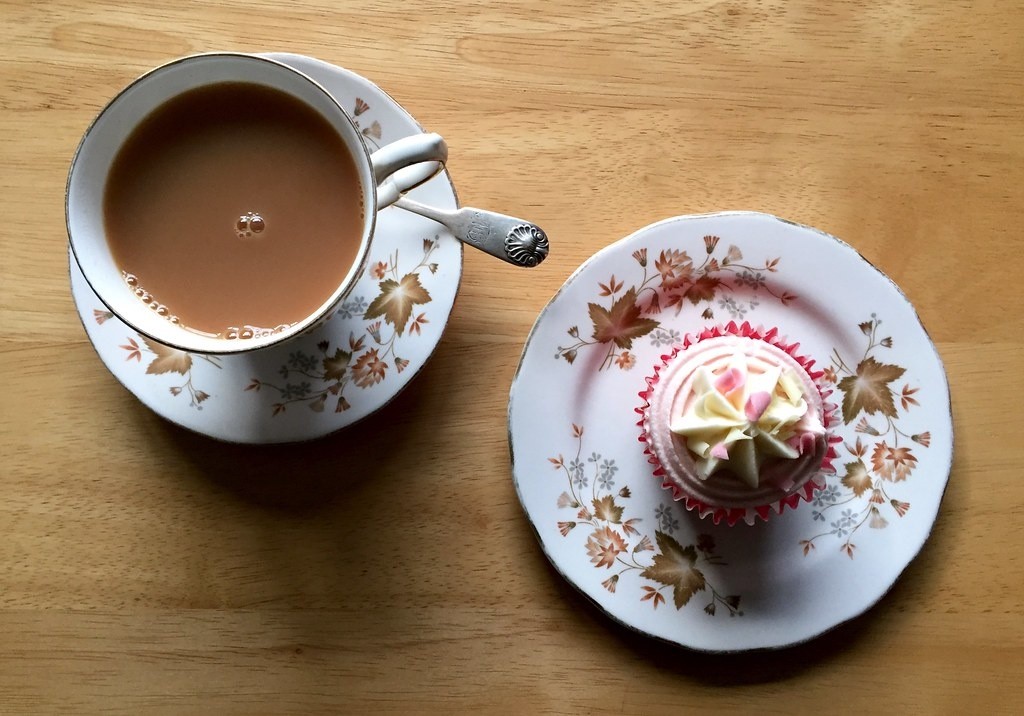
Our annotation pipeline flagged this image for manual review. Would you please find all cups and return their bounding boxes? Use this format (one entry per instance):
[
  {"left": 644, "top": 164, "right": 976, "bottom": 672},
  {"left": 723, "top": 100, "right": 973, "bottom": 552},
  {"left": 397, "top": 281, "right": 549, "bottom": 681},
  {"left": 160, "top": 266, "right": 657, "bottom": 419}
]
[{"left": 64, "top": 51, "right": 448, "bottom": 356}]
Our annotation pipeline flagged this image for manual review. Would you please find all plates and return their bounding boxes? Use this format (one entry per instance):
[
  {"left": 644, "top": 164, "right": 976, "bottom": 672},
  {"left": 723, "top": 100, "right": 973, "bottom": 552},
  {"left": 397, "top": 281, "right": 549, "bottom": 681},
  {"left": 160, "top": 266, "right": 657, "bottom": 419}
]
[
  {"left": 68, "top": 48, "right": 458, "bottom": 442},
  {"left": 506, "top": 212, "right": 955, "bottom": 652}
]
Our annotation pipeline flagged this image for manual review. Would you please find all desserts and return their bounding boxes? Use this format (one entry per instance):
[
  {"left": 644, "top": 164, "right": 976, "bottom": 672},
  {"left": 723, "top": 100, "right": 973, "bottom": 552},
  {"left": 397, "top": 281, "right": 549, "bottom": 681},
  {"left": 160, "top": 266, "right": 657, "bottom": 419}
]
[{"left": 636, "top": 323, "right": 841, "bottom": 525}]
